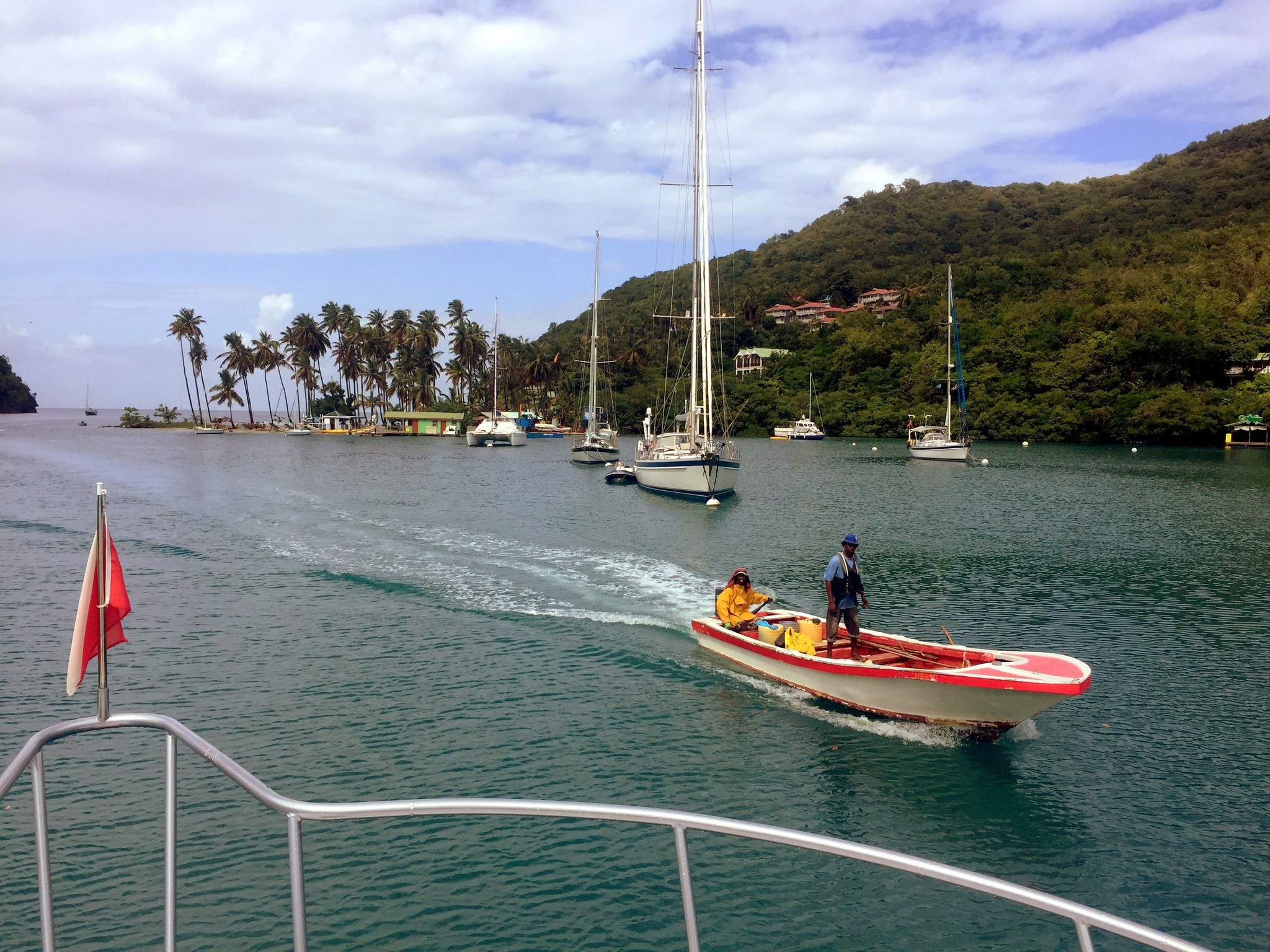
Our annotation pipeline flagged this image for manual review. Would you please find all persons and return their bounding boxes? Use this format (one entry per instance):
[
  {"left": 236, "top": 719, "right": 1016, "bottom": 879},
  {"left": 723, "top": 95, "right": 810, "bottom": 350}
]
[
  {"left": 824, "top": 533, "right": 871, "bottom": 661},
  {"left": 716, "top": 568, "right": 771, "bottom": 633}
]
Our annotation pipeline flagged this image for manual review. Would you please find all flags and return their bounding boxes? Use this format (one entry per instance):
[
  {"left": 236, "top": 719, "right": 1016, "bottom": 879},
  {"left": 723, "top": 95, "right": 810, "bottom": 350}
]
[{"left": 67, "top": 512, "right": 132, "bottom": 698}]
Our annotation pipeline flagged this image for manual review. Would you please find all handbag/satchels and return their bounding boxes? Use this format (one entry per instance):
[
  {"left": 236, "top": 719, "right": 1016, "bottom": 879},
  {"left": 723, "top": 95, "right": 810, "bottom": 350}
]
[{"left": 784, "top": 626, "right": 817, "bottom": 656}]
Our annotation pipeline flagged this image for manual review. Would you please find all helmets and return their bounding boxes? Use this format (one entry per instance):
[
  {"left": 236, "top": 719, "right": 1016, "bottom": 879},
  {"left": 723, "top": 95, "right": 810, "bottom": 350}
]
[{"left": 844, "top": 533, "right": 859, "bottom": 544}]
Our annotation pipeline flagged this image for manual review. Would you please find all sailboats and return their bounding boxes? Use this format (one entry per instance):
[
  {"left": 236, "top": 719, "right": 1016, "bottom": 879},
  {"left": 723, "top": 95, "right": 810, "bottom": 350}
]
[
  {"left": 774, "top": 372, "right": 826, "bottom": 441},
  {"left": 904, "top": 264, "right": 974, "bottom": 462},
  {"left": 571, "top": 229, "right": 622, "bottom": 465},
  {"left": 84, "top": 378, "right": 98, "bottom": 416},
  {"left": 466, "top": 297, "right": 528, "bottom": 447},
  {"left": 630, "top": 0, "right": 741, "bottom": 503},
  {"left": 192, "top": 388, "right": 225, "bottom": 434},
  {"left": 283, "top": 387, "right": 312, "bottom": 436}
]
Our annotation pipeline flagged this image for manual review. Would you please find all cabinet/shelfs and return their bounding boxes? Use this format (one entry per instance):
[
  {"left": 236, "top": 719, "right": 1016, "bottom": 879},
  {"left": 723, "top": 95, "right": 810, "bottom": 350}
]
[{"left": 717, "top": 614, "right": 796, "bottom": 627}]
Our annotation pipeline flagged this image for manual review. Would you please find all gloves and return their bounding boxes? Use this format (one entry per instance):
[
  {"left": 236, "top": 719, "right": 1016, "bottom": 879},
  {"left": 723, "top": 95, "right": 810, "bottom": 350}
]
[
  {"left": 724, "top": 622, "right": 732, "bottom": 629},
  {"left": 768, "top": 597, "right": 773, "bottom": 602}
]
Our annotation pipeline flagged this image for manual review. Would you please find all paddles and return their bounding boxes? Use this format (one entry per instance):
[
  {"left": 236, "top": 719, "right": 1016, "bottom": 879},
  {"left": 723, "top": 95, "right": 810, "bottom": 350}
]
[
  {"left": 941, "top": 625, "right": 954, "bottom": 646},
  {"left": 836, "top": 635, "right": 956, "bottom": 668}
]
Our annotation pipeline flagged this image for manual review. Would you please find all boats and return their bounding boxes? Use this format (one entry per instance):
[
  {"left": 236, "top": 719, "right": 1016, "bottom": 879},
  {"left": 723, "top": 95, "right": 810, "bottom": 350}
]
[
  {"left": 534, "top": 423, "right": 554, "bottom": 432},
  {"left": 269, "top": 421, "right": 286, "bottom": 432},
  {"left": 1223, "top": 413, "right": 1270, "bottom": 449},
  {"left": 690, "top": 607, "right": 1093, "bottom": 743},
  {"left": 302, "top": 422, "right": 320, "bottom": 433},
  {"left": 79, "top": 422, "right": 87, "bottom": 426},
  {"left": 605, "top": 461, "right": 636, "bottom": 484},
  {"left": 347, "top": 424, "right": 377, "bottom": 436},
  {"left": 557, "top": 427, "right": 572, "bottom": 433},
  {"left": 241, "top": 421, "right": 266, "bottom": 429}
]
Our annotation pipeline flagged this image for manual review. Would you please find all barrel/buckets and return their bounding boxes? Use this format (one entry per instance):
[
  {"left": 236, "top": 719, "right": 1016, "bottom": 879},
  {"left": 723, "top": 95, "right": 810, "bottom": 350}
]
[
  {"left": 758, "top": 623, "right": 784, "bottom": 646},
  {"left": 797, "top": 618, "right": 823, "bottom": 642},
  {"left": 779, "top": 621, "right": 798, "bottom": 632}
]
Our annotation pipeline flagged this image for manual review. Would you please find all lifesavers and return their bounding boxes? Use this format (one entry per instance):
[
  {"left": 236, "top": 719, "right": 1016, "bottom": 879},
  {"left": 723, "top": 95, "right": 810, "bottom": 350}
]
[{"left": 909, "top": 439, "right": 912, "bottom": 446}]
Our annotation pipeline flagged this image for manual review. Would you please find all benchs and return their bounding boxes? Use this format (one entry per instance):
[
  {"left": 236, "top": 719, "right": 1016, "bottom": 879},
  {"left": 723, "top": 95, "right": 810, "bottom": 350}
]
[
  {"left": 846, "top": 653, "right": 902, "bottom": 664},
  {"left": 811, "top": 639, "right": 851, "bottom": 649}
]
